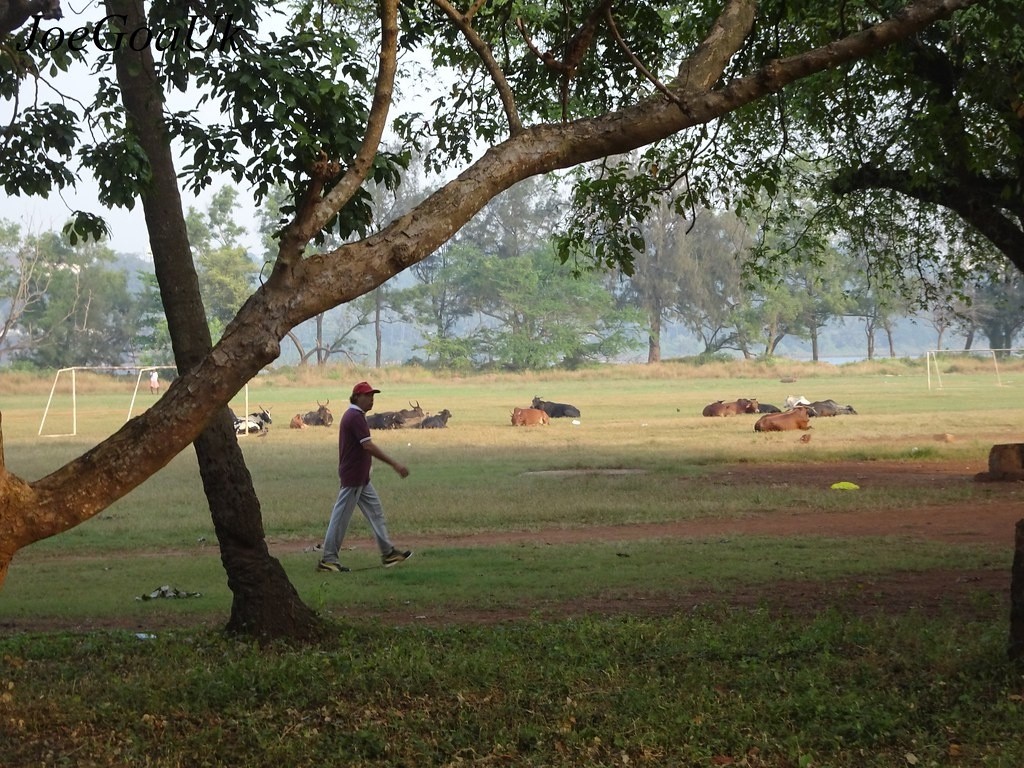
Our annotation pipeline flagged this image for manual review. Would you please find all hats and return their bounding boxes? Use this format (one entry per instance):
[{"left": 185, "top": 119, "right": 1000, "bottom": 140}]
[{"left": 354, "top": 382, "right": 380, "bottom": 395}]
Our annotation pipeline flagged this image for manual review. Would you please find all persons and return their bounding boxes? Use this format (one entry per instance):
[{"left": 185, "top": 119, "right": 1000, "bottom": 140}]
[{"left": 316, "top": 381, "right": 412, "bottom": 570}]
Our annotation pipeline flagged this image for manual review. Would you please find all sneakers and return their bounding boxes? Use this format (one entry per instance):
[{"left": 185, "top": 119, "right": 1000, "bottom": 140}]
[
  {"left": 317, "top": 561, "right": 352, "bottom": 573},
  {"left": 380, "top": 548, "right": 412, "bottom": 569}
]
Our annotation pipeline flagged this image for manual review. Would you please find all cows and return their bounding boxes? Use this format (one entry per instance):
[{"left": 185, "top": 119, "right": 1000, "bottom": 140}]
[
  {"left": 702, "top": 393, "right": 859, "bottom": 433},
  {"left": 529, "top": 395, "right": 581, "bottom": 418},
  {"left": 419, "top": 408, "right": 452, "bottom": 429},
  {"left": 508, "top": 406, "right": 549, "bottom": 426},
  {"left": 365, "top": 399, "right": 425, "bottom": 431},
  {"left": 232, "top": 404, "right": 273, "bottom": 437},
  {"left": 289, "top": 398, "right": 334, "bottom": 431}
]
